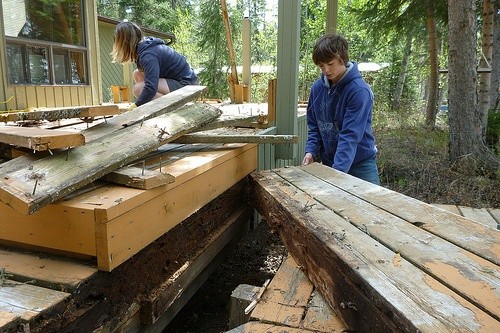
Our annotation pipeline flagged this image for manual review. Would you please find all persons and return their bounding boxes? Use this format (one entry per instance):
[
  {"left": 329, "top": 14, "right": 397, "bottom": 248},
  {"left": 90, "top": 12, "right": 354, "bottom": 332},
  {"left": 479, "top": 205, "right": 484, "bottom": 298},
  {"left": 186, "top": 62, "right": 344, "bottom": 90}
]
[
  {"left": 109, "top": 21, "right": 198, "bottom": 112},
  {"left": 300, "top": 33, "right": 381, "bottom": 186}
]
